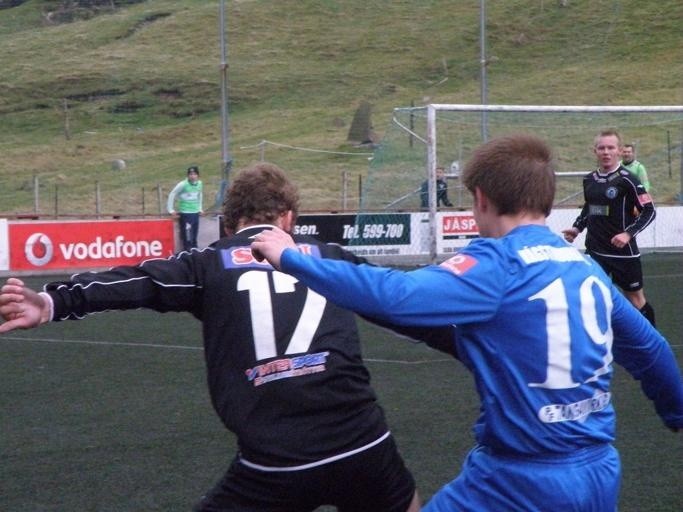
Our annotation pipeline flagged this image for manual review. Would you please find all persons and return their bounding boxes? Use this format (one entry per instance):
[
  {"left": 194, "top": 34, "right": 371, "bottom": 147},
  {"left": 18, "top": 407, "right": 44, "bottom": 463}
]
[
  {"left": 420, "top": 166, "right": 453, "bottom": 208},
  {"left": 618, "top": 142, "right": 650, "bottom": 215},
  {"left": 249, "top": 131, "right": 682, "bottom": 512},
  {"left": 166, "top": 166, "right": 205, "bottom": 253},
  {"left": 560, "top": 131, "right": 657, "bottom": 329},
  {"left": 1, "top": 159, "right": 460, "bottom": 511}
]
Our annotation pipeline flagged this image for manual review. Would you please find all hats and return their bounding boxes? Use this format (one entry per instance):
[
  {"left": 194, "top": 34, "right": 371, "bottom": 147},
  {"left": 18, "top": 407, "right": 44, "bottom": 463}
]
[{"left": 188, "top": 167, "right": 198, "bottom": 174}]
